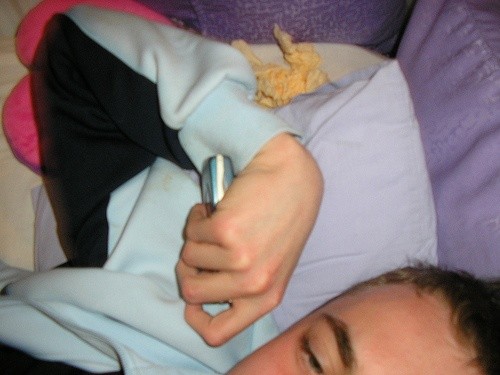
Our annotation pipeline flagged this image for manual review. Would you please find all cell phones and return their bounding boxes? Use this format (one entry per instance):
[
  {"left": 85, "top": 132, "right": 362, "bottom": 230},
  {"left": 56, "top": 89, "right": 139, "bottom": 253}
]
[{"left": 201, "top": 155, "right": 234, "bottom": 217}]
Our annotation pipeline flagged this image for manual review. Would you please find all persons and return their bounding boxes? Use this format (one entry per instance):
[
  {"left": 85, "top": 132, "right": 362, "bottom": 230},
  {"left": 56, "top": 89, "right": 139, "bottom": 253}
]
[{"left": 0, "top": 6, "right": 500, "bottom": 375}]
